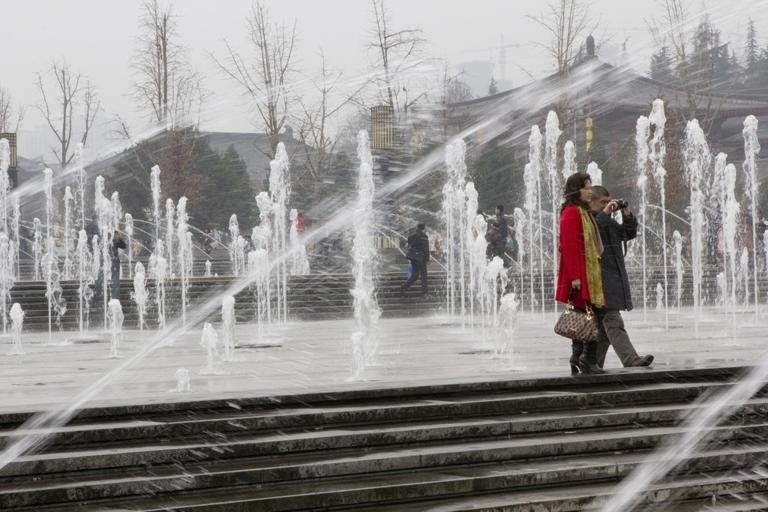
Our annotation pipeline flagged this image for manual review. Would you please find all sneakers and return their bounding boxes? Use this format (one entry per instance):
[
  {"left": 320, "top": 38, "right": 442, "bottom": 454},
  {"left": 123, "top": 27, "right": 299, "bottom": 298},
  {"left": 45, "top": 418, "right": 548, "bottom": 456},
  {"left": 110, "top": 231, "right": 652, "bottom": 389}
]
[{"left": 630, "top": 355, "right": 654, "bottom": 366}]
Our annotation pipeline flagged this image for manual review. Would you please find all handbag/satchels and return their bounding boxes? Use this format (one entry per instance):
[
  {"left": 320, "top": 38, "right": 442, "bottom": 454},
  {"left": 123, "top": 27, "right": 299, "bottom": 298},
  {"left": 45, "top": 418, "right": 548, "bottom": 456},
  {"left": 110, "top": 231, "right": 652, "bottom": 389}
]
[
  {"left": 405, "top": 247, "right": 424, "bottom": 262},
  {"left": 554, "top": 288, "right": 600, "bottom": 344}
]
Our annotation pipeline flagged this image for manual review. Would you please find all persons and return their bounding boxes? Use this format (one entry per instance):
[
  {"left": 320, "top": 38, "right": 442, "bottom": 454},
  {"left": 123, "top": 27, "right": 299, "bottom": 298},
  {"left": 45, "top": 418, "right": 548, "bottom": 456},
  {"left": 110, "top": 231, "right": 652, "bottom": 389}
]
[
  {"left": 443, "top": 204, "right": 525, "bottom": 268},
  {"left": 202, "top": 227, "right": 214, "bottom": 254},
  {"left": 84, "top": 214, "right": 127, "bottom": 299},
  {"left": 556, "top": 173, "right": 606, "bottom": 374},
  {"left": 589, "top": 185, "right": 654, "bottom": 366},
  {"left": 243, "top": 234, "right": 255, "bottom": 263},
  {"left": 400, "top": 223, "right": 441, "bottom": 298}
]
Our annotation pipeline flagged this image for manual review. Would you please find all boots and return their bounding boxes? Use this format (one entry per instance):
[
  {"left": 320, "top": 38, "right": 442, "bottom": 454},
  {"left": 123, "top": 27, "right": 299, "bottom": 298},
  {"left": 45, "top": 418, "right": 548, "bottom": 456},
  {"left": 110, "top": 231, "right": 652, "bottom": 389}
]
[{"left": 570, "top": 340, "right": 607, "bottom": 376}]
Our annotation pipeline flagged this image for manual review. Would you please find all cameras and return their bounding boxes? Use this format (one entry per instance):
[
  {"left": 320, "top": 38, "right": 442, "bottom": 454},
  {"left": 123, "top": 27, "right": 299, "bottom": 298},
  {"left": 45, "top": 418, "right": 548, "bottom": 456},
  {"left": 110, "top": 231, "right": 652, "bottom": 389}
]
[{"left": 611, "top": 200, "right": 628, "bottom": 211}]
